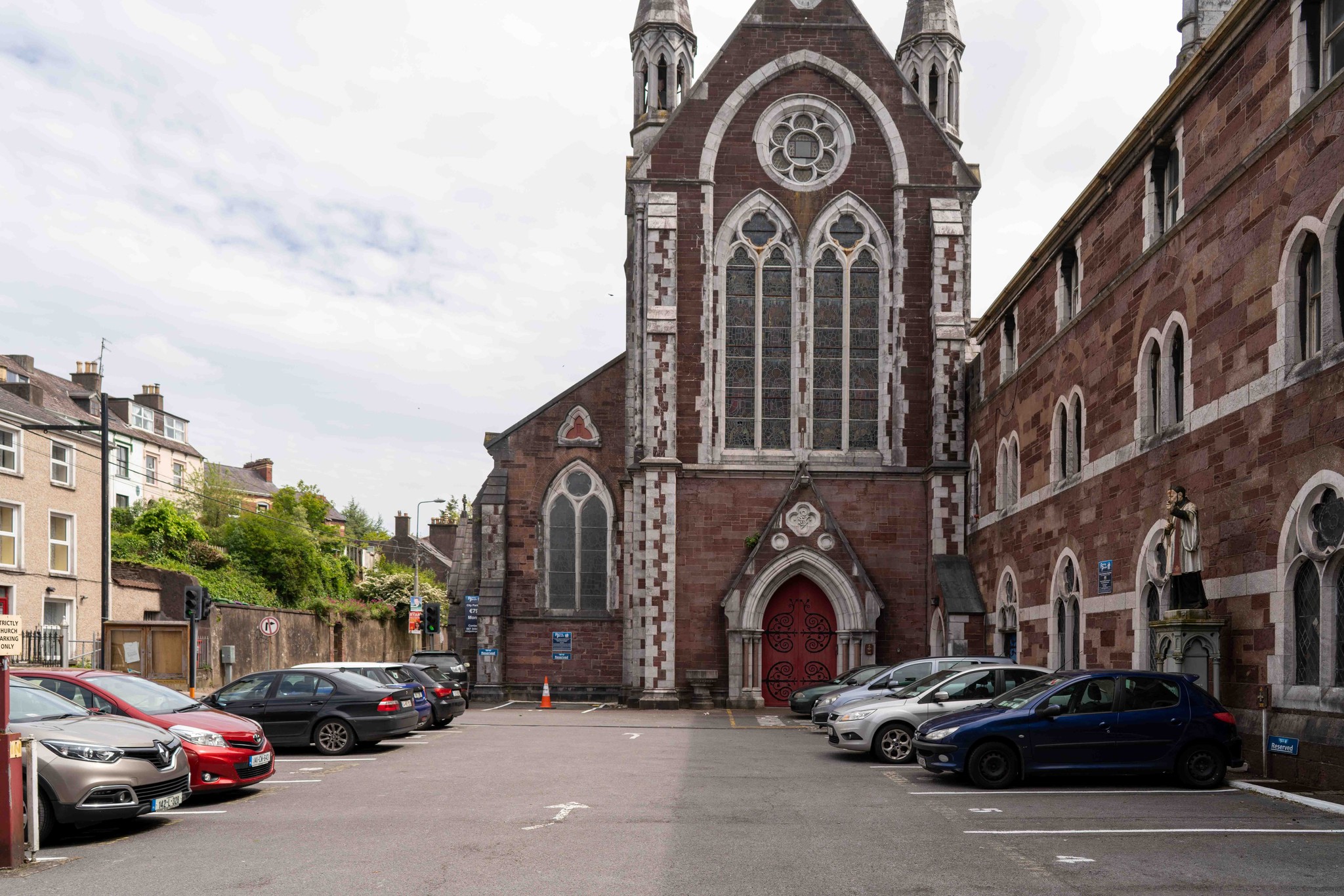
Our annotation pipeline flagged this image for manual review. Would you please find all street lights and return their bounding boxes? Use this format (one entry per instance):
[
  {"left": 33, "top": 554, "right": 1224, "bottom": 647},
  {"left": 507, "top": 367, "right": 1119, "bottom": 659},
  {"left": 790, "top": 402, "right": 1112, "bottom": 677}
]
[{"left": 412, "top": 499, "right": 446, "bottom": 652}]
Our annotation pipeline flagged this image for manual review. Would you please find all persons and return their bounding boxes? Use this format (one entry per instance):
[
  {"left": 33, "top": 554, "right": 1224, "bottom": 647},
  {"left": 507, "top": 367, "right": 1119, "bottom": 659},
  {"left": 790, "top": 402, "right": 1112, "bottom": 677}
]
[
  {"left": 413, "top": 597, "right": 421, "bottom": 607},
  {"left": 1162, "top": 484, "right": 1208, "bottom": 610},
  {"left": 413, "top": 621, "right": 421, "bottom": 631}
]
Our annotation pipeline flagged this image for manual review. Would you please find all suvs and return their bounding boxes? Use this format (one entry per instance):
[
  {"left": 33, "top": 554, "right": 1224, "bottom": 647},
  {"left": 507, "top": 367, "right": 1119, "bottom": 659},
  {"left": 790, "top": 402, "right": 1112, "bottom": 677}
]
[{"left": 409, "top": 648, "right": 471, "bottom": 708}]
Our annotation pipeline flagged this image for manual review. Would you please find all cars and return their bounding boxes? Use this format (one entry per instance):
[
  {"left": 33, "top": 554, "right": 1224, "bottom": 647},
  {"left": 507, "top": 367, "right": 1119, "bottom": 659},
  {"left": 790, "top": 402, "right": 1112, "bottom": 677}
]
[
  {"left": 193, "top": 668, "right": 420, "bottom": 756},
  {"left": 9, "top": 674, "right": 193, "bottom": 849},
  {"left": 228, "top": 660, "right": 432, "bottom": 727},
  {"left": 390, "top": 662, "right": 466, "bottom": 730},
  {"left": 814, "top": 656, "right": 1023, "bottom": 728},
  {"left": 827, "top": 664, "right": 1108, "bottom": 764},
  {"left": 8, "top": 666, "right": 277, "bottom": 792},
  {"left": 788, "top": 665, "right": 890, "bottom": 713},
  {"left": 911, "top": 668, "right": 1244, "bottom": 790}
]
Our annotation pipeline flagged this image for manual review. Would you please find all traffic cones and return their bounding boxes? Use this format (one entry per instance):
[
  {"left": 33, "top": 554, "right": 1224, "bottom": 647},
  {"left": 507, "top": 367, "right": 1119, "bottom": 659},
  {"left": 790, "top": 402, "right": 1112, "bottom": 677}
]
[{"left": 536, "top": 676, "right": 557, "bottom": 709}]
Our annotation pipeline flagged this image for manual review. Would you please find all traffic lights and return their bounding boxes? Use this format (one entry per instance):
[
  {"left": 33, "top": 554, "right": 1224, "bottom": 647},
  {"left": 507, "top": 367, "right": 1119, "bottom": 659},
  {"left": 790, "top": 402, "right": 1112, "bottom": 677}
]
[
  {"left": 425, "top": 603, "right": 440, "bottom": 634},
  {"left": 419, "top": 612, "right": 425, "bottom": 631},
  {"left": 202, "top": 587, "right": 211, "bottom": 620},
  {"left": 184, "top": 586, "right": 201, "bottom": 620}
]
